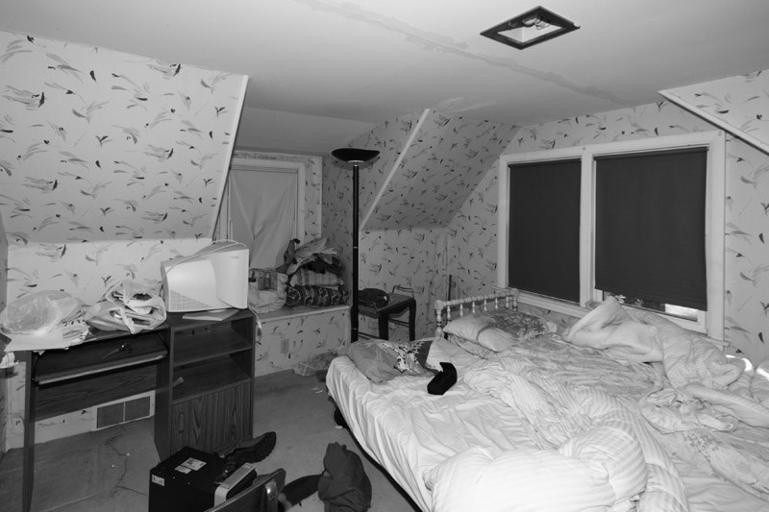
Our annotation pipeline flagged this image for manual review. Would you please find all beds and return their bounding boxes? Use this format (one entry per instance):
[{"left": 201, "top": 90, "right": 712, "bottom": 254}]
[{"left": 325, "top": 290, "right": 769, "bottom": 509}]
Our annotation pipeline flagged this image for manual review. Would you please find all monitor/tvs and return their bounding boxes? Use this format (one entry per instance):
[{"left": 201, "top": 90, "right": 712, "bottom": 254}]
[{"left": 160, "top": 240, "right": 251, "bottom": 322}]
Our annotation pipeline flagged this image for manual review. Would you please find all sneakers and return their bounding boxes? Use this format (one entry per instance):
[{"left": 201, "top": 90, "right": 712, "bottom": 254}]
[{"left": 215, "top": 430, "right": 278, "bottom": 486}]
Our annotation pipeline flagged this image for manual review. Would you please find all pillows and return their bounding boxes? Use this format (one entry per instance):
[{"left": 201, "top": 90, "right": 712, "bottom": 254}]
[{"left": 444, "top": 307, "right": 557, "bottom": 353}]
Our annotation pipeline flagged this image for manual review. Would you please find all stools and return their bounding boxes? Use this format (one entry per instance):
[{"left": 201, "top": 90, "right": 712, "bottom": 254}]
[{"left": 359, "top": 293, "right": 416, "bottom": 344}]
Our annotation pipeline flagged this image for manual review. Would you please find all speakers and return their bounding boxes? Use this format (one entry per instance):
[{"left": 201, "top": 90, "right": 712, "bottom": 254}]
[{"left": 148, "top": 446, "right": 256, "bottom": 512}]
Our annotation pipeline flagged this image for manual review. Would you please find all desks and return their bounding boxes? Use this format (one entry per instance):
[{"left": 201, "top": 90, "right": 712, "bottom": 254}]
[{"left": 1, "top": 308, "right": 256, "bottom": 510}]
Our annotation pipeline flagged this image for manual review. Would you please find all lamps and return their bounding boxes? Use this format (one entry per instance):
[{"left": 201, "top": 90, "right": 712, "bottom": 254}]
[{"left": 332, "top": 146, "right": 379, "bottom": 428}]
